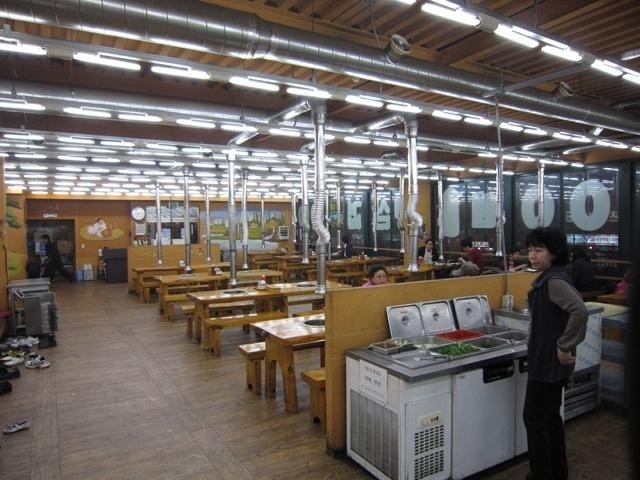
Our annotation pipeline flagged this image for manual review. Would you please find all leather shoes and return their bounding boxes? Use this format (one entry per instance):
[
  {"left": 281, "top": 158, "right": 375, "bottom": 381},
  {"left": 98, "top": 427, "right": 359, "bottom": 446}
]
[{"left": 2, "top": 419, "right": 31, "bottom": 434}]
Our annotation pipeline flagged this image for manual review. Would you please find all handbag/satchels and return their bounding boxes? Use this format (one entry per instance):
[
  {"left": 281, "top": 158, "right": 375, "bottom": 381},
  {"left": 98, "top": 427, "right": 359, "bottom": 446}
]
[{"left": 452, "top": 261, "right": 480, "bottom": 277}]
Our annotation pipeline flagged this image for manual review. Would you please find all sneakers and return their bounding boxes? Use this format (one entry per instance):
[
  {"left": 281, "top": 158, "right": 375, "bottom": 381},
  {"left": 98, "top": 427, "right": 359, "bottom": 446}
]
[{"left": 0, "top": 335, "right": 50, "bottom": 395}]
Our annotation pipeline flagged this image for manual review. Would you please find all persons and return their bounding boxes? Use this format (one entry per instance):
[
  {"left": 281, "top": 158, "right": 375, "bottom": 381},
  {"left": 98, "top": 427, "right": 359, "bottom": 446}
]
[
  {"left": 462, "top": 237, "right": 484, "bottom": 276},
  {"left": 41, "top": 233, "right": 74, "bottom": 283},
  {"left": 525, "top": 227, "right": 588, "bottom": 480},
  {"left": 568, "top": 247, "right": 595, "bottom": 291},
  {"left": 341, "top": 237, "right": 352, "bottom": 259},
  {"left": 416, "top": 237, "right": 440, "bottom": 262},
  {"left": 360, "top": 267, "right": 389, "bottom": 287},
  {"left": 501, "top": 251, "right": 528, "bottom": 272}
]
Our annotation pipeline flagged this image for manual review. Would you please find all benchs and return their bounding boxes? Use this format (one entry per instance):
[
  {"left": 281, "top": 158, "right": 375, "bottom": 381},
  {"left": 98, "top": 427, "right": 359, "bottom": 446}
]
[
  {"left": 298, "top": 368, "right": 327, "bottom": 433},
  {"left": 130, "top": 247, "right": 462, "bottom": 415}
]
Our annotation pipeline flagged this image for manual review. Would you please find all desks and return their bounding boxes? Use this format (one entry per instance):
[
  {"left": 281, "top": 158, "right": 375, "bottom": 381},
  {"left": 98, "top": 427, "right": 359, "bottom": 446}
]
[{"left": 7, "top": 277, "right": 52, "bottom": 338}]
